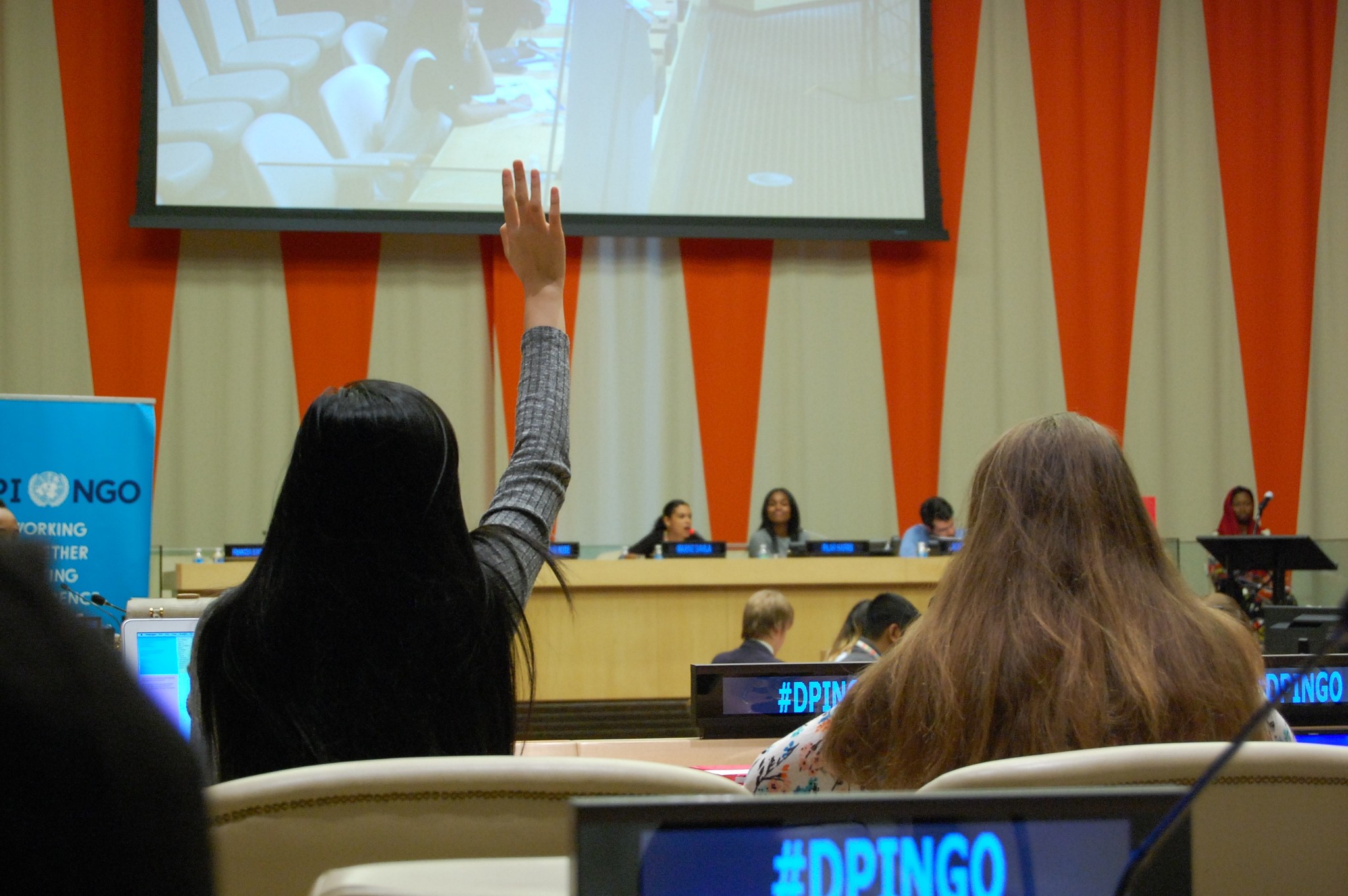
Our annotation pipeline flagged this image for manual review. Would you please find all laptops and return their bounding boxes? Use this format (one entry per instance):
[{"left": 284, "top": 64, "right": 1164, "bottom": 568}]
[{"left": 120, "top": 617, "right": 201, "bottom": 741}]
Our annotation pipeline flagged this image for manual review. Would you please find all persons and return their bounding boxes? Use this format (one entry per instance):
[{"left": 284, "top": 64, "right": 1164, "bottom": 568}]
[
  {"left": 473, "top": 0, "right": 543, "bottom": 53},
  {"left": 839, "top": 592, "right": 922, "bottom": 662},
  {"left": 748, "top": 487, "right": 813, "bottom": 557},
  {"left": 380, "top": 0, "right": 531, "bottom": 124},
  {"left": 1208, "top": 484, "right": 1298, "bottom": 642},
  {"left": 0, "top": 509, "right": 212, "bottom": 896},
  {"left": 737, "top": 411, "right": 1299, "bottom": 794},
  {"left": 184, "top": 159, "right": 570, "bottom": 784},
  {"left": 898, "top": 496, "right": 965, "bottom": 556},
  {"left": 710, "top": 587, "right": 795, "bottom": 664},
  {"left": 627, "top": 499, "right": 705, "bottom": 558},
  {"left": 825, "top": 599, "right": 871, "bottom": 662},
  {"left": 1201, "top": 593, "right": 1250, "bottom": 632}
]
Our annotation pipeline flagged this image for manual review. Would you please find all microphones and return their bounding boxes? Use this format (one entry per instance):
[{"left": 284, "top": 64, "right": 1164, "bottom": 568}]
[
  {"left": 688, "top": 528, "right": 705, "bottom": 541},
  {"left": 1257, "top": 488, "right": 1273, "bottom": 517},
  {"left": 62, "top": 583, "right": 120, "bottom": 629},
  {"left": 91, "top": 593, "right": 127, "bottom": 614},
  {"left": 797, "top": 526, "right": 826, "bottom": 540}
]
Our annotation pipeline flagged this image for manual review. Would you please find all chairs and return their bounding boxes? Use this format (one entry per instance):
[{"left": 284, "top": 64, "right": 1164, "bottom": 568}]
[
  {"left": 203, "top": 759, "right": 749, "bottom": 896},
  {"left": 914, "top": 736, "right": 1348, "bottom": 896}
]
